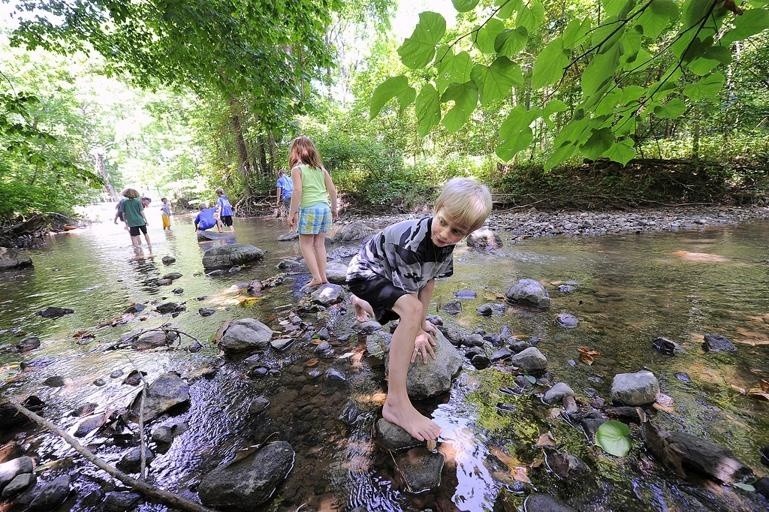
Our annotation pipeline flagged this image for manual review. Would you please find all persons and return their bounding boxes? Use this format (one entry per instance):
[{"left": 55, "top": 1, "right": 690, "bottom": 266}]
[
  {"left": 287, "top": 136, "right": 338, "bottom": 288},
  {"left": 160, "top": 197, "right": 172, "bottom": 232},
  {"left": 275, "top": 169, "right": 299, "bottom": 233},
  {"left": 114, "top": 188, "right": 153, "bottom": 245},
  {"left": 193, "top": 200, "right": 223, "bottom": 232},
  {"left": 345, "top": 176, "right": 492, "bottom": 443},
  {"left": 215, "top": 187, "right": 236, "bottom": 233}
]
